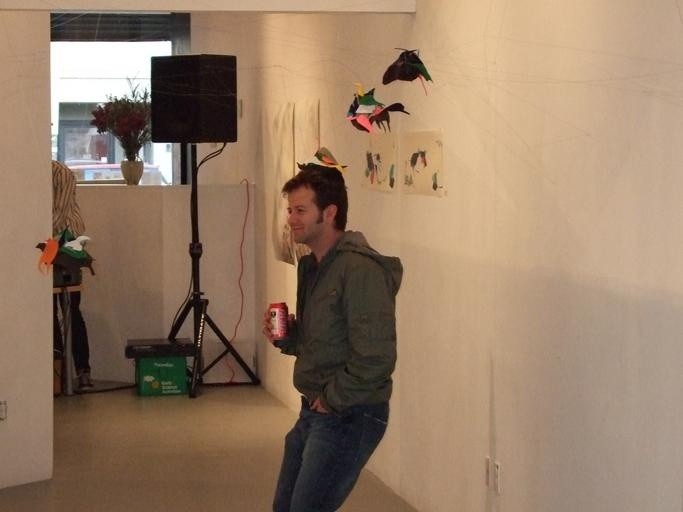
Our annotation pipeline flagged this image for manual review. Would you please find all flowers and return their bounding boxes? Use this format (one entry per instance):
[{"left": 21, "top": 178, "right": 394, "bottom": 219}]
[{"left": 90, "top": 73, "right": 152, "bottom": 162}]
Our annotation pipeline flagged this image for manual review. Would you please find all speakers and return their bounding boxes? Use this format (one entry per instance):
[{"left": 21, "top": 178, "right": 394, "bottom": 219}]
[{"left": 150, "top": 54, "right": 238, "bottom": 143}]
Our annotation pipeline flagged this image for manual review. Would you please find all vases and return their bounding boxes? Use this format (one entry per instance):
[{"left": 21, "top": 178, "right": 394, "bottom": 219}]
[{"left": 120, "top": 160, "right": 145, "bottom": 185}]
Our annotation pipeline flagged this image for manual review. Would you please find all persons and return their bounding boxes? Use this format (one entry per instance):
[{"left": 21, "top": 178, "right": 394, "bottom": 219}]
[
  {"left": 260, "top": 165, "right": 405, "bottom": 512},
  {"left": 52, "top": 159, "right": 95, "bottom": 397}
]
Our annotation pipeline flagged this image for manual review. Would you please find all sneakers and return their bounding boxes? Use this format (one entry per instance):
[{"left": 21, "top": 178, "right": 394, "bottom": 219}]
[{"left": 78, "top": 374, "right": 94, "bottom": 391}]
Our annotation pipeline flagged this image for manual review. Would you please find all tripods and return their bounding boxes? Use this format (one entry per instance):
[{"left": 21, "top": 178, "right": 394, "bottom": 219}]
[{"left": 167, "top": 144, "right": 260, "bottom": 399}]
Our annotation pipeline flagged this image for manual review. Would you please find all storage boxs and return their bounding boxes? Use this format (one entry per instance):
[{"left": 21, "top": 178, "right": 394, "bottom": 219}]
[{"left": 134, "top": 357, "right": 188, "bottom": 395}]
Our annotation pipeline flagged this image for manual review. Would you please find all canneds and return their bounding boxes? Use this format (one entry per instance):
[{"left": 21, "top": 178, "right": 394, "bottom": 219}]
[{"left": 268, "top": 302, "right": 288, "bottom": 339}]
